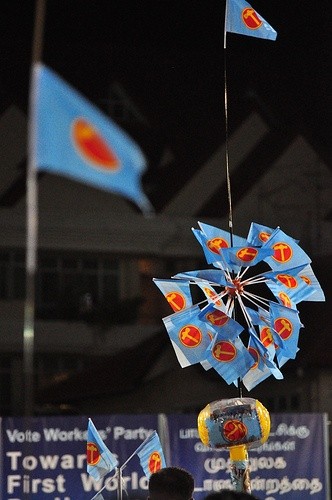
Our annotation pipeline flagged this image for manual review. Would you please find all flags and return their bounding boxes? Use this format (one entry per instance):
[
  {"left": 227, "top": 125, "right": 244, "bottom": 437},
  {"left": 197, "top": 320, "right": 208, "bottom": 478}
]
[
  {"left": 152, "top": 220, "right": 327, "bottom": 392},
  {"left": 29, "top": 62, "right": 153, "bottom": 217},
  {"left": 225, "top": 0, "right": 278, "bottom": 41},
  {"left": 86, "top": 418, "right": 117, "bottom": 482},
  {"left": 136, "top": 430, "right": 166, "bottom": 482}
]
